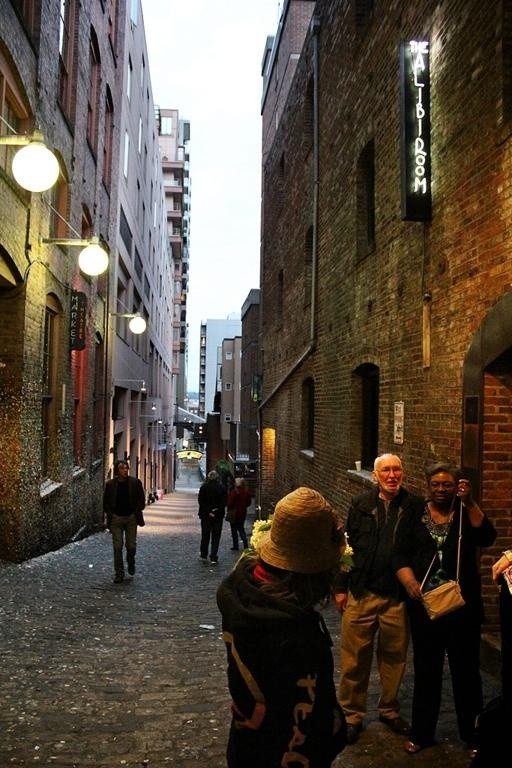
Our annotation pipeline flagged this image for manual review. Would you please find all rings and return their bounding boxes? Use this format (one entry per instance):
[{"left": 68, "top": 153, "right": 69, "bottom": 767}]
[{"left": 460, "top": 487, "right": 464, "bottom": 492}]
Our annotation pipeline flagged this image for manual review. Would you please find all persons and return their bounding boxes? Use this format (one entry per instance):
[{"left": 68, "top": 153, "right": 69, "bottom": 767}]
[
  {"left": 102, "top": 457, "right": 144, "bottom": 585},
  {"left": 197, "top": 469, "right": 227, "bottom": 566},
  {"left": 223, "top": 477, "right": 252, "bottom": 551},
  {"left": 215, "top": 481, "right": 353, "bottom": 768},
  {"left": 389, "top": 462, "right": 499, "bottom": 758},
  {"left": 220, "top": 467, "right": 236, "bottom": 518},
  {"left": 490, "top": 546, "right": 511, "bottom": 582},
  {"left": 331, "top": 450, "right": 422, "bottom": 746}
]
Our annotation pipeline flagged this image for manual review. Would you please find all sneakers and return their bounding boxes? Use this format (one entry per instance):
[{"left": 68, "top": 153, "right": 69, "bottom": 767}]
[
  {"left": 231, "top": 547, "right": 238, "bottom": 549},
  {"left": 200, "top": 557, "right": 216, "bottom": 566}
]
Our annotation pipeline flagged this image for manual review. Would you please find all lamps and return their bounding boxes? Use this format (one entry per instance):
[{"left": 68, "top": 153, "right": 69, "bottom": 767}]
[
  {"left": 39, "top": 231, "right": 109, "bottom": 276},
  {"left": 109, "top": 310, "right": 147, "bottom": 335},
  {"left": 0, "top": 126, "right": 60, "bottom": 193}
]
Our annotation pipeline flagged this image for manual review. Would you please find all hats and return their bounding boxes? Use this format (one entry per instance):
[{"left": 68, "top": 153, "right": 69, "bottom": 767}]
[{"left": 258, "top": 488, "right": 347, "bottom": 575}]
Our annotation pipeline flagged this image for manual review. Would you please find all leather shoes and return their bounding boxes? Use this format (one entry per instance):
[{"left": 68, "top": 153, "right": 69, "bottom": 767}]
[
  {"left": 128, "top": 569, "right": 134, "bottom": 575},
  {"left": 380, "top": 715, "right": 412, "bottom": 735},
  {"left": 344, "top": 723, "right": 363, "bottom": 745},
  {"left": 114, "top": 576, "right": 123, "bottom": 583},
  {"left": 468, "top": 748, "right": 480, "bottom": 757},
  {"left": 404, "top": 741, "right": 422, "bottom": 753}
]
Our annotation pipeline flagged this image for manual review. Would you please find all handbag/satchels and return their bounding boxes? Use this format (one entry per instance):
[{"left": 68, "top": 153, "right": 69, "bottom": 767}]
[
  {"left": 423, "top": 581, "right": 465, "bottom": 618},
  {"left": 225, "top": 509, "right": 236, "bottom": 522}
]
